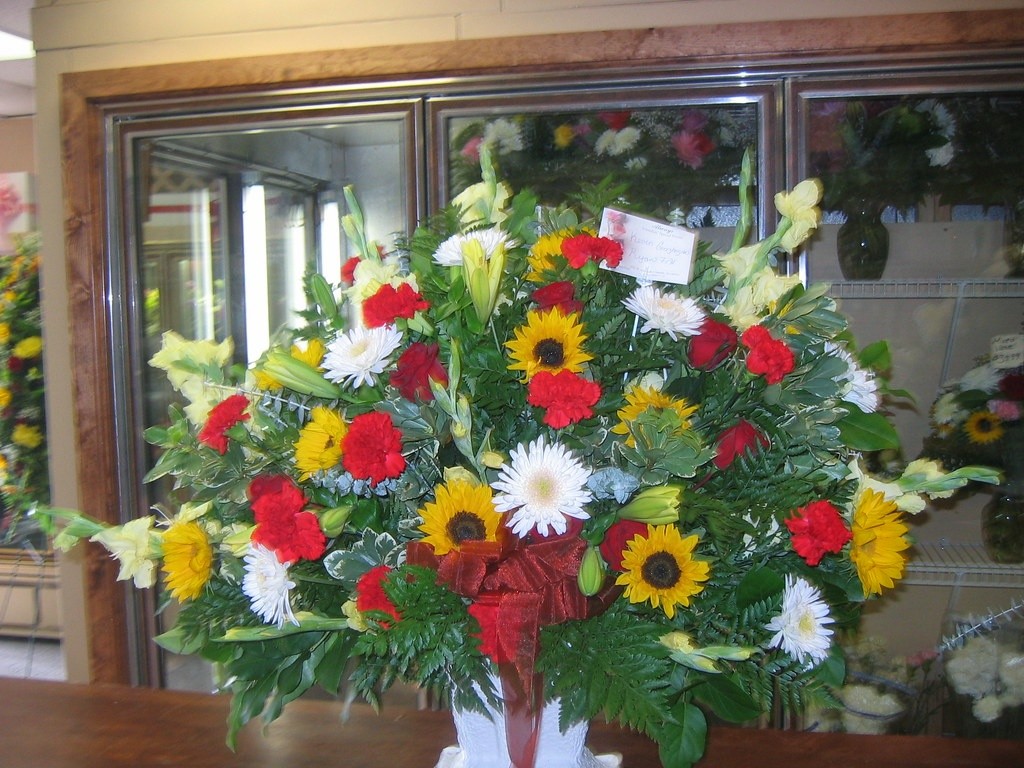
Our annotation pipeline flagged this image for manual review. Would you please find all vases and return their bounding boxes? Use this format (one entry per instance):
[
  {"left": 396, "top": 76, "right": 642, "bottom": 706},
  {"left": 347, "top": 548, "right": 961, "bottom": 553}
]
[
  {"left": 982, "top": 477, "right": 1024, "bottom": 569},
  {"left": 837, "top": 198, "right": 889, "bottom": 284}
]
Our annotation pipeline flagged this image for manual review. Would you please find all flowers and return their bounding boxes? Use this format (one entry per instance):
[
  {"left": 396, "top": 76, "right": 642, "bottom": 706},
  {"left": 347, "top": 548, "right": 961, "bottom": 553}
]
[
  {"left": 76, "top": 120, "right": 929, "bottom": 762},
  {"left": 792, "top": 93, "right": 956, "bottom": 217},
  {"left": 945, "top": 635, "right": 1024, "bottom": 725},
  {"left": 934, "top": 332, "right": 1022, "bottom": 490},
  {"left": 448, "top": 103, "right": 758, "bottom": 211},
  {"left": 1, "top": 227, "right": 53, "bottom": 548}
]
